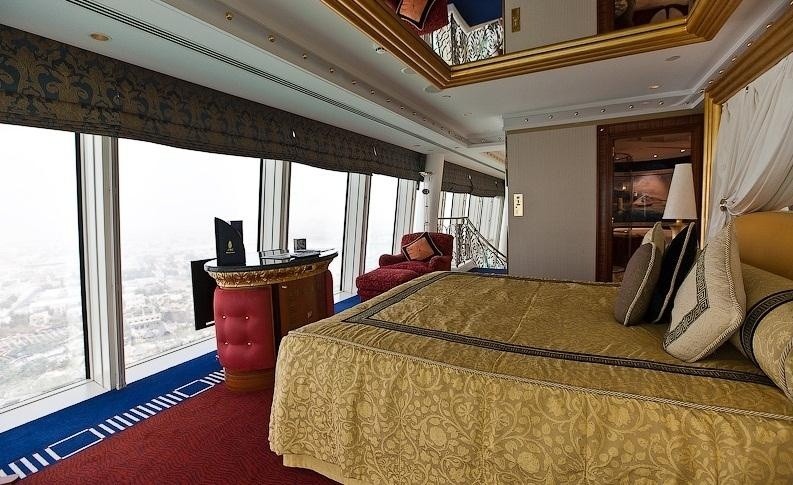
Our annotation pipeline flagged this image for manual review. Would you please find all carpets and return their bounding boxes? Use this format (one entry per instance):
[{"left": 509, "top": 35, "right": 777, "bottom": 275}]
[{"left": 13, "top": 380, "right": 344, "bottom": 485}]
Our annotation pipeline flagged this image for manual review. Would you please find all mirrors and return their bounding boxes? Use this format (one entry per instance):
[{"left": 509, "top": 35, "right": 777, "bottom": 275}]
[{"left": 321, "top": 0, "right": 742, "bottom": 92}]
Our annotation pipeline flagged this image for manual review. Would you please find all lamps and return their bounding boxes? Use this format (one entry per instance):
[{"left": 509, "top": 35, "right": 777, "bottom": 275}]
[{"left": 661, "top": 163, "right": 698, "bottom": 240}]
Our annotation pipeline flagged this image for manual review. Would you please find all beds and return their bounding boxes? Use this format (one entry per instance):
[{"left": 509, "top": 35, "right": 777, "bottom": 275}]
[{"left": 268, "top": 211, "right": 793, "bottom": 485}]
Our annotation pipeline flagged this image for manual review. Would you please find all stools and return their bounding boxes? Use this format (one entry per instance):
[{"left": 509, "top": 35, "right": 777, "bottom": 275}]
[{"left": 356, "top": 268, "right": 420, "bottom": 302}]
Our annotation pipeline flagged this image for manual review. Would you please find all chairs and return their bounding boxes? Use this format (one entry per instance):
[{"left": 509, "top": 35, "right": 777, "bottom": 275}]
[
  {"left": 384, "top": 0, "right": 449, "bottom": 36},
  {"left": 378, "top": 232, "right": 454, "bottom": 276}
]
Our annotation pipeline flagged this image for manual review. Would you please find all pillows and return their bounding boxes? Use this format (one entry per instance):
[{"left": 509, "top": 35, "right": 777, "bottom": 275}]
[
  {"left": 613, "top": 220, "right": 793, "bottom": 404},
  {"left": 394, "top": 0, "right": 436, "bottom": 32},
  {"left": 402, "top": 231, "right": 443, "bottom": 263}
]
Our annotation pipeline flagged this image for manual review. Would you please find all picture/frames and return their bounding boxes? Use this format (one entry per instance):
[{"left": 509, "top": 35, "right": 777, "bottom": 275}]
[{"left": 612, "top": 169, "right": 692, "bottom": 227}]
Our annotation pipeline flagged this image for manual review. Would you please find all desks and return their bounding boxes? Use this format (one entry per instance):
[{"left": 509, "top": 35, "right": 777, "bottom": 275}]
[{"left": 203, "top": 247, "right": 339, "bottom": 393}]
